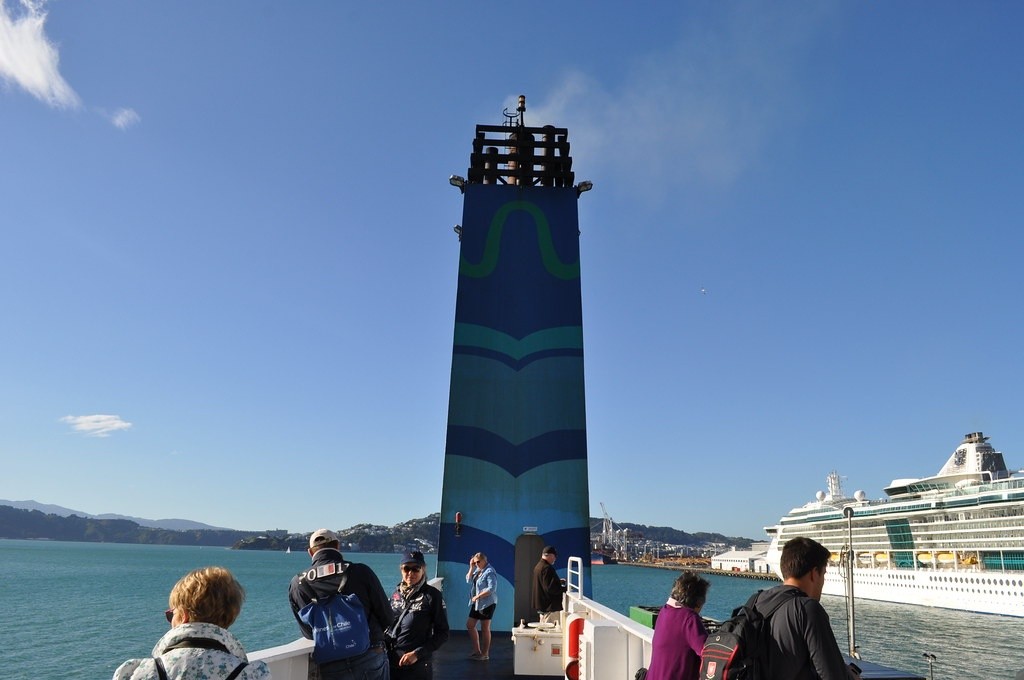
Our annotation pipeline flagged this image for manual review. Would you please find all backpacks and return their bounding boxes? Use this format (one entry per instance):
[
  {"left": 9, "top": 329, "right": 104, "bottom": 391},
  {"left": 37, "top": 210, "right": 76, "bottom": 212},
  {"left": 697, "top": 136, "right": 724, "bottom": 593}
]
[
  {"left": 296, "top": 563, "right": 371, "bottom": 665},
  {"left": 698, "top": 589, "right": 809, "bottom": 680}
]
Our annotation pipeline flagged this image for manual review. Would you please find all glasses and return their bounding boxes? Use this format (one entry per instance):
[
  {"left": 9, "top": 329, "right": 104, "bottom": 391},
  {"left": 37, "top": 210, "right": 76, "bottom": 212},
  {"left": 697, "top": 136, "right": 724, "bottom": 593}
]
[
  {"left": 473, "top": 560, "right": 480, "bottom": 565},
  {"left": 404, "top": 565, "right": 421, "bottom": 572},
  {"left": 164, "top": 607, "right": 177, "bottom": 622}
]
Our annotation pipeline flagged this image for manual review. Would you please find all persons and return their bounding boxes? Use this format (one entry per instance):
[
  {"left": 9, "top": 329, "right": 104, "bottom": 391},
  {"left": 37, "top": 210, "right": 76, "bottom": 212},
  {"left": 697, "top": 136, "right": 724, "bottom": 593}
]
[
  {"left": 466, "top": 552, "right": 497, "bottom": 659},
  {"left": 532, "top": 546, "right": 567, "bottom": 624},
  {"left": 112, "top": 566, "right": 272, "bottom": 680},
  {"left": 383, "top": 551, "right": 449, "bottom": 680},
  {"left": 645, "top": 571, "right": 710, "bottom": 680},
  {"left": 288, "top": 529, "right": 395, "bottom": 680},
  {"left": 738, "top": 536, "right": 859, "bottom": 680}
]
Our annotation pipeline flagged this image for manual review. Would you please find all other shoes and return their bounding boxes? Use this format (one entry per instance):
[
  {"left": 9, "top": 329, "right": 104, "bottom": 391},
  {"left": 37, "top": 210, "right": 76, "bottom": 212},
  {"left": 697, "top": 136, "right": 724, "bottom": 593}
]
[
  {"left": 473, "top": 653, "right": 489, "bottom": 661},
  {"left": 468, "top": 649, "right": 481, "bottom": 658}
]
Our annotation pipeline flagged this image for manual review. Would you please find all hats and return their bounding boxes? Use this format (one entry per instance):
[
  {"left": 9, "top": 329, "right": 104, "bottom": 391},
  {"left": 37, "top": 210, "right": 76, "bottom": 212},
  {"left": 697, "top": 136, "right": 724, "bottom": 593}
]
[
  {"left": 401, "top": 550, "right": 425, "bottom": 564},
  {"left": 309, "top": 529, "right": 339, "bottom": 549},
  {"left": 542, "top": 546, "right": 558, "bottom": 554}
]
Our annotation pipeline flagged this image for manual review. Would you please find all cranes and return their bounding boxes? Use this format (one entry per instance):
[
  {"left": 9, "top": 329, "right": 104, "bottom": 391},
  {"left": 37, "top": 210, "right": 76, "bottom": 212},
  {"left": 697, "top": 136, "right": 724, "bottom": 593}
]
[{"left": 599, "top": 502, "right": 628, "bottom": 562}]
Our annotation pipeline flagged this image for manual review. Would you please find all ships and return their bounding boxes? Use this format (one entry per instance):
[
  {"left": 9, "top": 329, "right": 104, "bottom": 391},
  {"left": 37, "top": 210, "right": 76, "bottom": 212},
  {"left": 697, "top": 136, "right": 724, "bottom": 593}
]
[{"left": 764, "top": 432, "right": 1024, "bottom": 617}]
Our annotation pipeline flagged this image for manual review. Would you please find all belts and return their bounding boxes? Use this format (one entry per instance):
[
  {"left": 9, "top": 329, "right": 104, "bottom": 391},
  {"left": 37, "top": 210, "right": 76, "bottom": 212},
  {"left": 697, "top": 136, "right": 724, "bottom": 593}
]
[{"left": 321, "top": 653, "right": 370, "bottom": 673}]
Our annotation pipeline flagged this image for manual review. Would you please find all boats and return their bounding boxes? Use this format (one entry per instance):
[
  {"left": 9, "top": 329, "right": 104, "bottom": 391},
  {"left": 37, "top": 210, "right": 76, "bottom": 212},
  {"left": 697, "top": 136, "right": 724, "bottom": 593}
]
[
  {"left": 918, "top": 554, "right": 939, "bottom": 564},
  {"left": 858, "top": 553, "right": 873, "bottom": 565},
  {"left": 938, "top": 553, "right": 960, "bottom": 564},
  {"left": 829, "top": 555, "right": 840, "bottom": 562},
  {"left": 875, "top": 553, "right": 888, "bottom": 562}
]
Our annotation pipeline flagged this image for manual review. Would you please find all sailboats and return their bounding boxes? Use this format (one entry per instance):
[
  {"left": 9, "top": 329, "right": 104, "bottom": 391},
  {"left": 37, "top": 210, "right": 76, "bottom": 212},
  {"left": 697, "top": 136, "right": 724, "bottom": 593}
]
[{"left": 286, "top": 547, "right": 290, "bottom": 553}]
